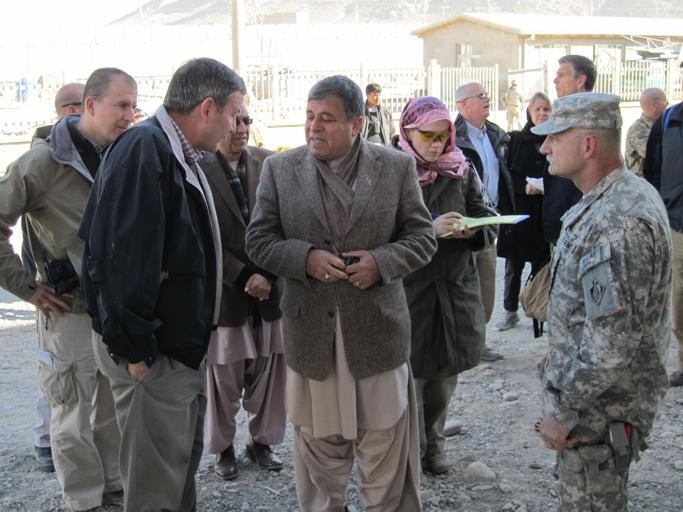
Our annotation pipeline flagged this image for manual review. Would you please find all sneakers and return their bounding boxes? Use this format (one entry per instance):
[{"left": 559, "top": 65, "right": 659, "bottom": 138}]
[
  {"left": 35, "top": 446, "right": 55, "bottom": 472},
  {"left": 483, "top": 348, "right": 503, "bottom": 361},
  {"left": 670, "top": 371, "right": 683, "bottom": 386},
  {"left": 495, "top": 311, "right": 519, "bottom": 329},
  {"left": 427, "top": 458, "right": 451, "bottom": 473}
]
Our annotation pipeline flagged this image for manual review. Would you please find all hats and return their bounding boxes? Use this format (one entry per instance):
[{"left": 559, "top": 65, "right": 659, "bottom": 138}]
[{"left": 530, "top": 92, "right": 622, "bottom": 136}]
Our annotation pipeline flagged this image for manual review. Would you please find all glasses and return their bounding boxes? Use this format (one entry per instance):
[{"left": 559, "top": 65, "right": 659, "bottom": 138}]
[
  {"left": 236, "top": 119, "right": 253, "bottom": 125},
  {"left": 456, "top": 92, "right": 489, "bottom": 104},
  {"left": 415, "top": 127, "right": 451, "bottom": 142}
]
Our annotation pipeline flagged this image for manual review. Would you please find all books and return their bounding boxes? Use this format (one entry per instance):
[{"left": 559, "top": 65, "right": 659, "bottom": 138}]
[{"left": 439, "top": 213, "right": 531, "bottom": 237}]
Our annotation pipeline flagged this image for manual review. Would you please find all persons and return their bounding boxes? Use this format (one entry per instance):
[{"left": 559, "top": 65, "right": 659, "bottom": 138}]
[
  {"left": 197, "top": 98, "right": 288, "bottom": 478},
  {"left": 362, "top": 82, "right": 397, "bottom": 146},
  {"left": 1, "top": 67, "right": 139, "bottom": 512},
  {"left": 76, "top": 58, "right": 247, "bottom": 512},
  {"left": 20, "top": 81, "right": 87, "bottom": 474},
  {"left": 391, "top": 95, "right": 502, "bottom": 474},
  {"left": 641, "top": 102, "right": 682, "bottom": 387},
  {"left": 452, "top": 81, "right": 520, "bottom": 360},
  {"left": 245, "top": 76, "right": 439, "bottom": 510},
  {"left": 624, "top": 86, "right": 670, "bottom": 176},
  {"left": 496, "top": 90, "right": 555, "bottom": 330},
  {"left": 527, "top": 91, "right": 672, "bottom": 510},
  {"left": 526, "top": 53, "right": 599, "bottom": 259}
]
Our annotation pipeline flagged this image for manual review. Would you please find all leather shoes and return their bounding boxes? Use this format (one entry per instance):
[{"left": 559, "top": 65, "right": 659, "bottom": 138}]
[
  {"left": 245, "top": 440, "right": 282, "bottom": 470},
  {"left": 214, "top": 442, "right": 238, "bottom": 480}
]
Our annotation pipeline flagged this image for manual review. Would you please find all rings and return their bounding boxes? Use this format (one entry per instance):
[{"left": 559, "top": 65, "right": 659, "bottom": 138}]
[
  {"left": 354, "top": 280, "right": 363, "bottom": 288},
  {"left": 324, "top": 271, "right": 331, "bottom": 281}
]
